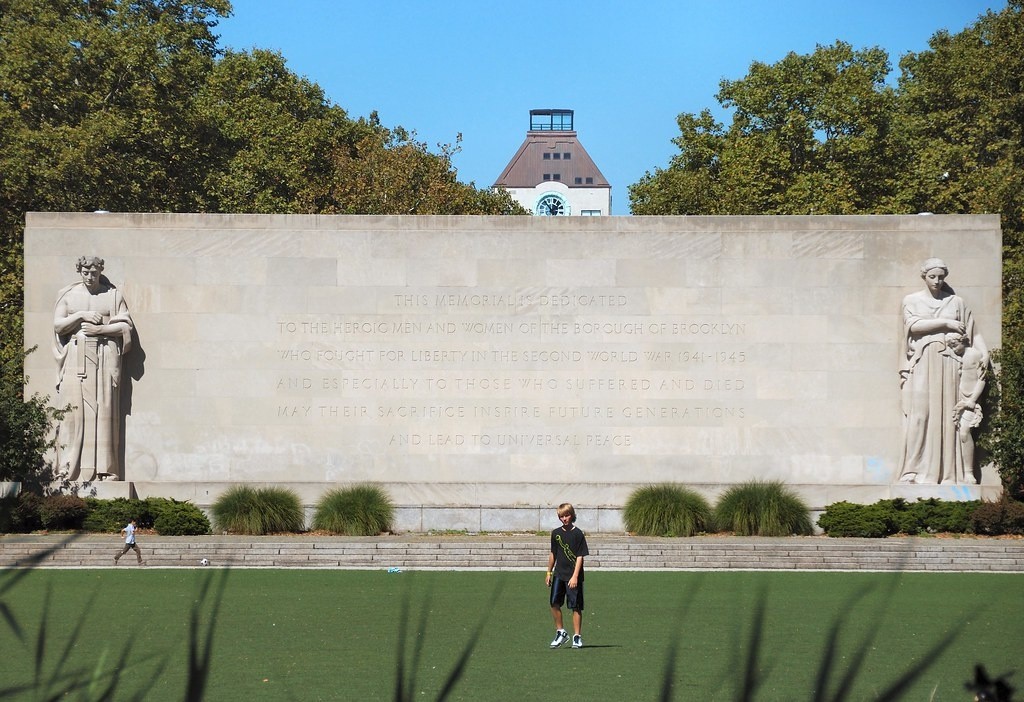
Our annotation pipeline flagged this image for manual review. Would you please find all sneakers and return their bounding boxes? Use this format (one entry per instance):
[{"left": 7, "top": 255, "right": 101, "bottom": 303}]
[
  {"left": 550, "top": 629, "right": 570, "bottom": 649},
  {"left": 572, "top": 634, "right": 582, "bottom": 649}
]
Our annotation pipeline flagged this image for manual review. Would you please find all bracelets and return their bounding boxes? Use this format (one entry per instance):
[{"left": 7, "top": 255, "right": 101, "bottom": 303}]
[{"left": 547, "top": 572, "right": 552, "bottom": 576}]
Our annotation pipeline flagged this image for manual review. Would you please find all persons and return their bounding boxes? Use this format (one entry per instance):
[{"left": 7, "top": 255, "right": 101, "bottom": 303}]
[
  {"left": 892, "top": 258, "right": 991, "bottom": 485},
  {"left": 545, "top": 503, "right": 589, "bottom": 649},
  {"left": 52, "top": 255, "right": 136, "bottom": 484},
  {"left": 113, "top": 518, "right": 146, "bottom": 566}
]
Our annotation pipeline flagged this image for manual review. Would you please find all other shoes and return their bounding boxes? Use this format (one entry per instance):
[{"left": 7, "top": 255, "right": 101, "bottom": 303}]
[
  {"left": 139, "top": 561, "right": 146, "bottom": 566},
  {"left": 113, "top": 558, "right": 117, "bottom": 566}
]
